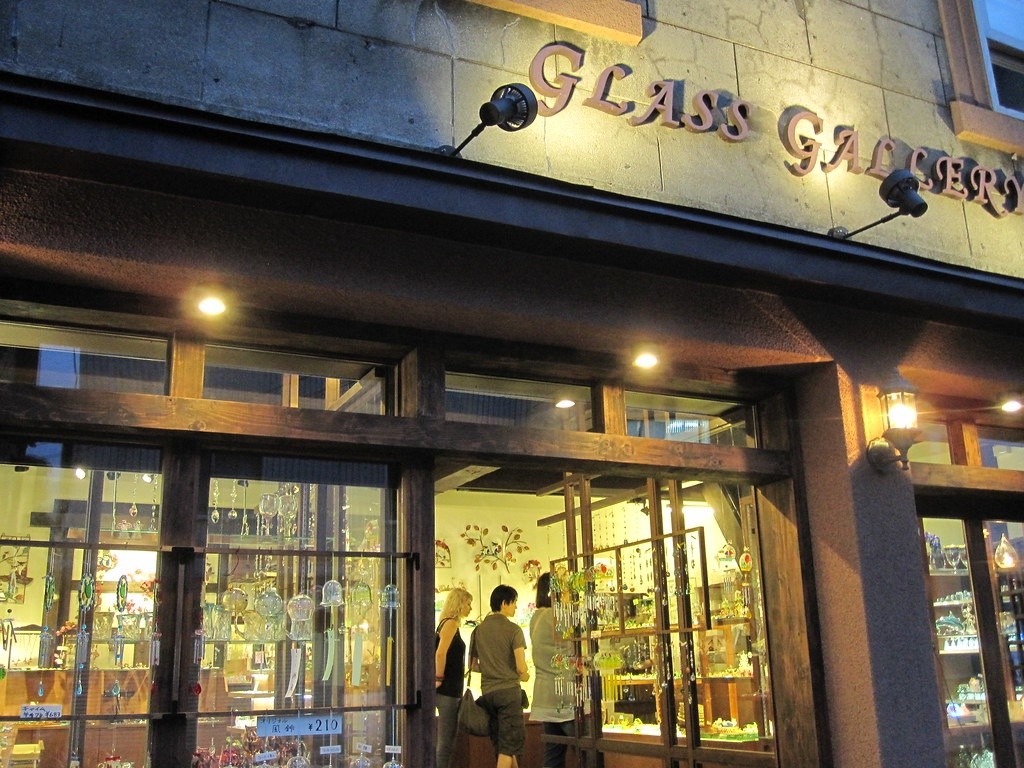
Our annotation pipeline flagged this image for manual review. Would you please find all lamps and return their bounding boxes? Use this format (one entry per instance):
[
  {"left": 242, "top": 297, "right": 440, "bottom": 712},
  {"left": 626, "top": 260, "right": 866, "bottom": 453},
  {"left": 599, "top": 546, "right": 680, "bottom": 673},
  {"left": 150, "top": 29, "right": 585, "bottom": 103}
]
[
  {"left": 994, "top": 532, "right": 1024, "bottom": 683},
  {"left": 827, "top": 170, "right": 927, "bottom": 241},
  {"left": 431, "top": 82, "right": 538, "bottom": 158},
  {"left": 864, "top": 366, "right": 923, "bottom": 477}
]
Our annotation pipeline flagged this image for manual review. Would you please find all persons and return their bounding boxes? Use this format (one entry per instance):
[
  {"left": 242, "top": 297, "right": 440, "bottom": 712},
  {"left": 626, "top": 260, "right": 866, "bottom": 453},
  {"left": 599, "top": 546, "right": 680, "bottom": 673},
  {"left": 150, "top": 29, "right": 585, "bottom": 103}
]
[
  {"left": 529, "top": 572, "right": 575, "bottom": 768},
  {"left": 468, "top": 584, "right": 531, "bottom": 768},
  {"left": 435, "top": 588, "right": 474, "bottom": 768}
]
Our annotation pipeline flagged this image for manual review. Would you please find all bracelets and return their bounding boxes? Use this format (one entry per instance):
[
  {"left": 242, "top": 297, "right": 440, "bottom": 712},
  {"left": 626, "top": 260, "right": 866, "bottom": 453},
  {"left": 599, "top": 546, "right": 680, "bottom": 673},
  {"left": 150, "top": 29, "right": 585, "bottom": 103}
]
[{"left": 435, "top": 675, "right": 444, "bottom": 682}]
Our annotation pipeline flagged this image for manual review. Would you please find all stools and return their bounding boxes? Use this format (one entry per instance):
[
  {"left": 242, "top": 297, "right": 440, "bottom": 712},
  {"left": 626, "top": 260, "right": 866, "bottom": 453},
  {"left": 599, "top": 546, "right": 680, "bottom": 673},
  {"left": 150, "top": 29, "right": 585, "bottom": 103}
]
[{"left": 9, "top": 740, "right": 44, "bottom": 768}]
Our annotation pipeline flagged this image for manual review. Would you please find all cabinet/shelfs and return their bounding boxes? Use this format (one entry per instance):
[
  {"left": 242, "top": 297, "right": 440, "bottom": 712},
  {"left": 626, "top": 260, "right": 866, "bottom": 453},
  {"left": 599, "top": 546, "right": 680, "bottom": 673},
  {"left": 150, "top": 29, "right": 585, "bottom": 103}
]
[
  {"left": 593, "top": 618, "right": 753, "bottom": 748},
  {"left": 929, "top": 569, "right": 1024, "bottom": 768}
]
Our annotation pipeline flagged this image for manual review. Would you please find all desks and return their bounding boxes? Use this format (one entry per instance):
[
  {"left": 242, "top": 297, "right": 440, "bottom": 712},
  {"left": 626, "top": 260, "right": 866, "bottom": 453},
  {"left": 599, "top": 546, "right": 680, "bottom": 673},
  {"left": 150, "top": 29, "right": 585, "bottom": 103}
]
[{"left": 0, "top": 668, "right": 227, "bottom": 716}]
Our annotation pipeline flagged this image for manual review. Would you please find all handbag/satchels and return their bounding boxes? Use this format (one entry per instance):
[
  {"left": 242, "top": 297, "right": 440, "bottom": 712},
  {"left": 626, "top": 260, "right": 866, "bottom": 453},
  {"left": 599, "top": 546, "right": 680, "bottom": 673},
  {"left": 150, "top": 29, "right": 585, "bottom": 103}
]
[{"left": 457, "top": 690, "right": 490, "bottom": 736}]
